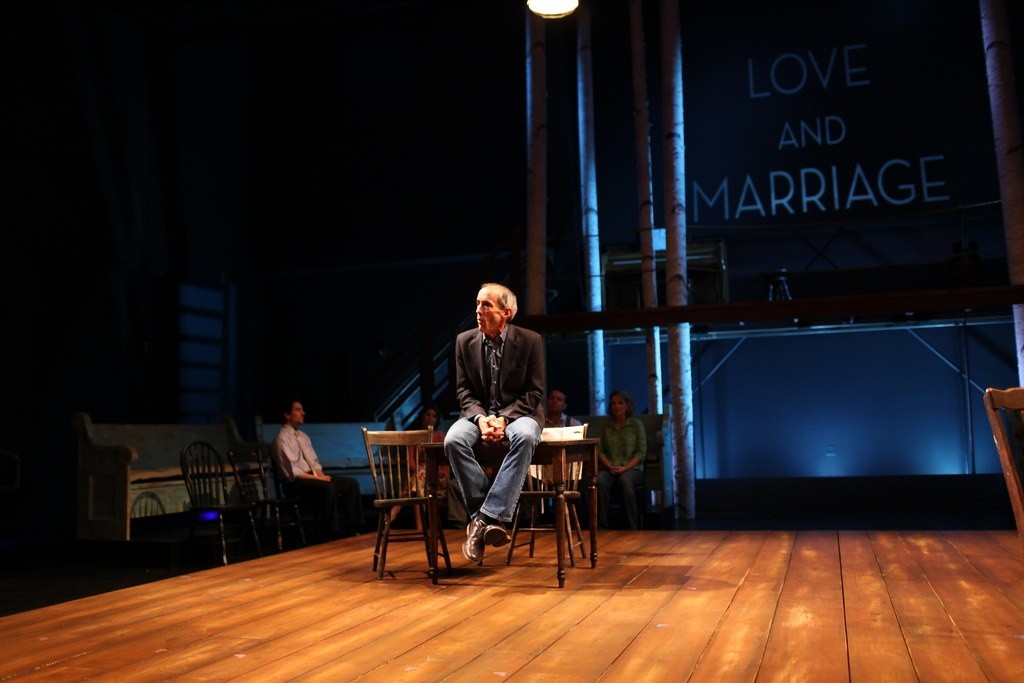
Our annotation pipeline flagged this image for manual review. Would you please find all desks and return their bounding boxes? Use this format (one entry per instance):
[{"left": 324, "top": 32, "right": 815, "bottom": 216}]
[{"left": 422, "top": 438, "right": 602, "bottom": 587}]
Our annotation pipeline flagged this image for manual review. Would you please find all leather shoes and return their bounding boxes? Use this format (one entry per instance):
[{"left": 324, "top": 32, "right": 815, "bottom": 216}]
[
  {"left": 462, "top": 517, "right": 486, "bottom": 564},
  {"left": 485, "top": 525, "right": 513, "bottom": 548}
]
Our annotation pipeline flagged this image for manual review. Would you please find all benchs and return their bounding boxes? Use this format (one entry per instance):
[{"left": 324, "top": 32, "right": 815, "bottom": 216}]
[
  {"left": 74, "top": 413, "right": 271, "bottom": 543},
  {"left": 576, "top": 414, "right": 673, "bottom": 530}
]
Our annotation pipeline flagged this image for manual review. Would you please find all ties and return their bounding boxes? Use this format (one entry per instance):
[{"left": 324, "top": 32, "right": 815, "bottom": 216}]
[{"left": 295, "top": 431, "right": 318, "bottom": 476}]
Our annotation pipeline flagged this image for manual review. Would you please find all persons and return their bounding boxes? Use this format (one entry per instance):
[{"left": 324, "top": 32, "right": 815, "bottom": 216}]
[
  {"left": 269, "top": 399, "right": 360, "bottom": 546},
  {"left": 598, "top": 389, "right": 649, "bottom": 529},
  {"left": 383, "top": 404, "right": 451, "bottom": 530},
  {"left": 443, "top": 283, "right": 546, "bottom": 565},
  {"left": 521, "top": 387, "right": 585, "bottom": 528}
]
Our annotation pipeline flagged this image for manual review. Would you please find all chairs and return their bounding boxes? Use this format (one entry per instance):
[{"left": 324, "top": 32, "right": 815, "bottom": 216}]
[
  {"left": 178, "top": 439, "right": 353, "bottom": 565},
  {"left": 984, "top": 387, "right": 1024, "bottom": 551},
  {"left": 506, "top": 423, "right": 589, "bottom": 568},
  {"left": 362, "top": 426, "right": 453, "bottom": 580}
]
